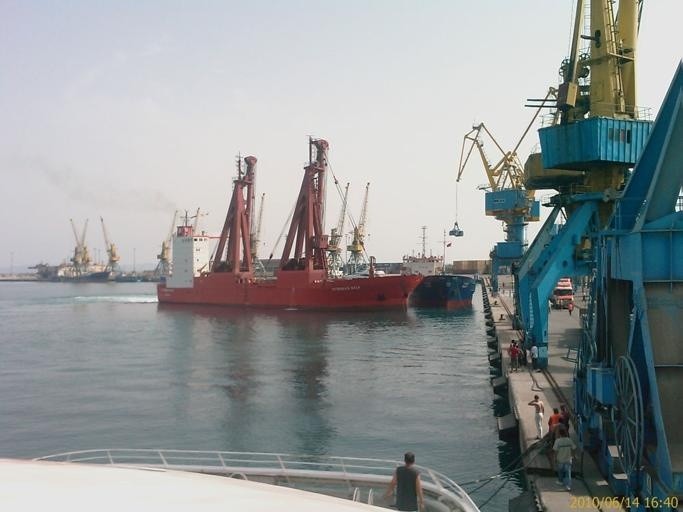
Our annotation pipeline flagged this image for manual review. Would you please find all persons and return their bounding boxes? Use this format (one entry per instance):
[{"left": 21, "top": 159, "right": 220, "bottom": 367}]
[
  {"left": 580, "top": 292, "right": 586, "bottom": 302},
  {"left": 528, "top": 394, "right": 544, "bottom": 438},
  {"left": 547, "top": 404, "right": 576, "bottom": 491},
  {"left": 568, "top": 302, "right": 574, "bottom": 316},
  {"left": 559, "top": 299, "right": 562, "bottom": 311},
  {"left": 507, "top": 339, "right": 539, "bottom": 373},
  {"left": 382, "top": 452, "right": 425, "bottom": 512},
  {"left": 548, "top": 300, "right": 553, "bottom": 314},
  {"left": 555, "top": 299, "right": 559, "bottom": 309}
]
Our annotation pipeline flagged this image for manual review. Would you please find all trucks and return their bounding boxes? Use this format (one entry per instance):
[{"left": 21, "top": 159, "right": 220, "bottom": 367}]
[{"left": 550, "top": 278, "right": 574, "bottom": 310}]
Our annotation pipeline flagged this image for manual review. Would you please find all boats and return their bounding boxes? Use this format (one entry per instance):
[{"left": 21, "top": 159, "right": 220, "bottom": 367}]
[
  {"left": 154, "top": 138, "right": 425, "bottom": 313},
  {"left": 0, "top": 446, "right": 480, "bottom": 512},
  {"left": 410, "top": 227, "right": 478, "bottom": 311},
  {"left": 27, "top": 214, "right": 172, "bottom": 283}
]
